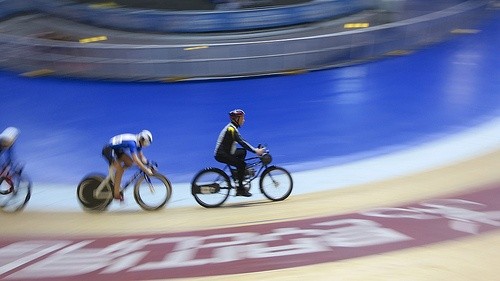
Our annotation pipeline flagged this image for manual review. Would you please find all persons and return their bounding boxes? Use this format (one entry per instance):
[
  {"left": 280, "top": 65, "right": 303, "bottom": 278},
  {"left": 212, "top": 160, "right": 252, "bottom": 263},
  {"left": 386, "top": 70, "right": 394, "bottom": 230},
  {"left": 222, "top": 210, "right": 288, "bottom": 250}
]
[
  {"left": 102, "top": 129, "right": 155, "bottom": 199},
  {"left": 213, "top": 109, "right": 264, "bottom": 197},
  {"left": 0, "top": 126, "right": 25, "bottom": 196}
]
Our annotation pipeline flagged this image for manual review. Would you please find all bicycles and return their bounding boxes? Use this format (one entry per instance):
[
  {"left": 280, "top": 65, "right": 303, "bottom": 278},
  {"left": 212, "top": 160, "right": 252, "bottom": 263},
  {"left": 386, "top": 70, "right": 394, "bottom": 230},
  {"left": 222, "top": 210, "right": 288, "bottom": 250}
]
[
  {"left": 0, "top": 162, "right": 32, "bottom": 214},
  {"left": 190, "top": 143, "right": 294, "bottom": 209},
  {"left": 76, "top": 160, "right": 173, "bottom": 212}
]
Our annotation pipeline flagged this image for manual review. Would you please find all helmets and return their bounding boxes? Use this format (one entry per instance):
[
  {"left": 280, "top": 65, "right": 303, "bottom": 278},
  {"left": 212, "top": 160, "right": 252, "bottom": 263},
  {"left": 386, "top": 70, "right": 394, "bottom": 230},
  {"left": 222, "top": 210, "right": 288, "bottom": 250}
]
[
  {"left": 139, "top": 129, "right": 153, "bottom": 146},
  {"left": 228, "top": 109, "right": 245, "bottom": 116}
]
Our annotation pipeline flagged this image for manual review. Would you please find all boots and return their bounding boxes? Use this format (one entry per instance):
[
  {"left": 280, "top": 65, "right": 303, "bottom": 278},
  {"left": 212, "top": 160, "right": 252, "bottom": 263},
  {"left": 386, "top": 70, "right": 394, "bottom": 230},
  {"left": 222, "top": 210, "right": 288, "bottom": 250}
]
[{"left": 236, "top": 186, "right": 252, "bottom": 197}]
